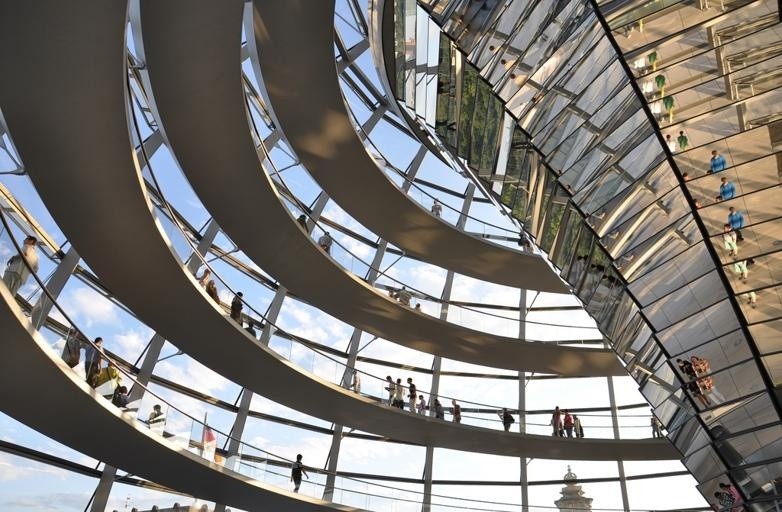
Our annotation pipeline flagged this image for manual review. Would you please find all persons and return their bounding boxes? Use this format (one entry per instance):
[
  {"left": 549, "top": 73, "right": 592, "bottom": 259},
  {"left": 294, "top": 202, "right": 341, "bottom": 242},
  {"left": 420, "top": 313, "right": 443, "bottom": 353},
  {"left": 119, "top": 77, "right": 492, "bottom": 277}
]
[
  {"left": 551, "top": 406, "right": 584, "bottom": 438},
  {"left": 713, "top": 479, "right": 744, "bottom": 511},
  {"left": 490, "top": 44, "right": 545, "bottom": 105},
  {"left": 679, "top": 151, "right": 757, "bottom": 306},
  {"left": 497, "top": 407, "right": 511, "bottom": 432},
  {"left": 519, "top": 227, "right": 534, "bottom": 254},
  {"left": 577, "top": 252, "right": 623, "bottom": 292},
  {"left": 432, "top": 399, "right": 462, "bottom": 424},
  {"left": 200, "top": 269, "right": 256, "bottom": 337},
  {"left": 62, "top": 327, "right": 81, "bottom": 369},
  {"left": 297, "top": 214, "right": 309, "bottom": 233},
  {"left": 432, "top": 197, "right": 442, "bottom": 217},
  {"left": 149, "top": 404, "right": 164, "bottom": 422},
  {"left": 291, "top": 454, "right": 309, "bottom": 494},
  {"left": 318, "top": 231, "right": 331, "bottom": 255},
  {"left": 677, "top": 354, "right": 725, "bottom": 406},
  {"left": 85, "top": 338, "right": 129, "bottom": 411},
  {"left": 390, "top": 286, "right": 422, "bottom": 312},
  {"left": 622, "top": 18, "right": 690, "bottom": 152},
  {"left": 650, "top": 416, "right": 662, "bottom": 440},
  {"left": 384, "top": 376, "right": 426, "bottom": 415},
  {"left": 351, "top": 370, "right": 360, "bottom": 393},
  {"left": 4, "top": 236, "right": 39, "bottom": 304}
]
